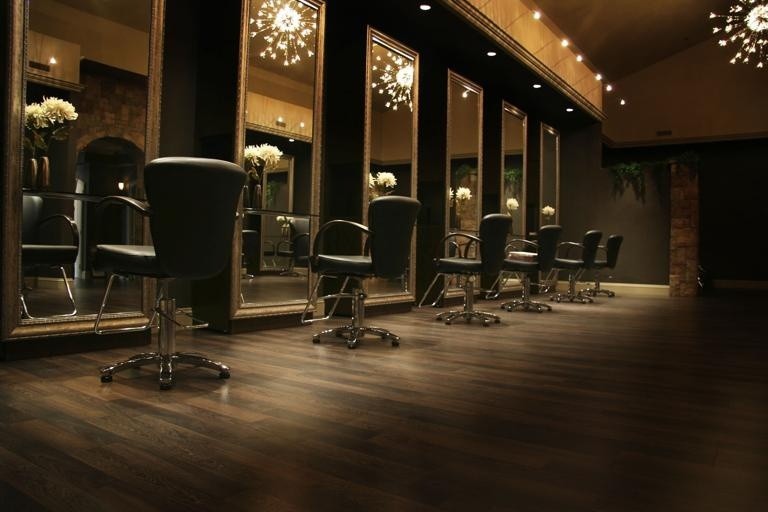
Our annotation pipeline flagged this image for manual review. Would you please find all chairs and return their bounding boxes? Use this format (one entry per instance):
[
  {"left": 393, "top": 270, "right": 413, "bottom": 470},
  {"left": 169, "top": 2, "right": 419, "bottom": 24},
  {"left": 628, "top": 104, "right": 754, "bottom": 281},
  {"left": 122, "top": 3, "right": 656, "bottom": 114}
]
[
  {"left": 537, "top": 226, "right": 603, "bottom": 308},
  {"left": 296, "top": 187, "right": 423, "bottom": 353},
  {"left": 578, "top": 233, "right": 624, "bottom": 300},
  {"left": 91, "top": 151, "right": 252, "bottom": 392}
]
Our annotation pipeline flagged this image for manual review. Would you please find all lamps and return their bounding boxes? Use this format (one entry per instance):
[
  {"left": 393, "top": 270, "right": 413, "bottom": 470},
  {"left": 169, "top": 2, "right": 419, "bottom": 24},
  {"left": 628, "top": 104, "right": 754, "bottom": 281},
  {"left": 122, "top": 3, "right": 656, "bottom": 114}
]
[{"left": 703, "top": 1, "right": 768, "bottom": 77}]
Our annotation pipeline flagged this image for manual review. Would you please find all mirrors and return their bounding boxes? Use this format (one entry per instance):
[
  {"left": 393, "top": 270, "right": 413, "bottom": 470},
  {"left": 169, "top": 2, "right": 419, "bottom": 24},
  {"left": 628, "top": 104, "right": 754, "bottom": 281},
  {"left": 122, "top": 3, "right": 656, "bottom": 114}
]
[
  {"left": 446, "top": 67, "right": 484, "bottom": 300},
  {"left": 539, "top": 122, "right": 559, "bottom": 287},
  {"left": 1, "top": 0, "right": 164, "bottom": 337},
  {"left": 500, "top": 99, "right": 531, "bottom": 293},
  {"left": 228, "top": 0, "right": 327, "bottom": 319},
  {"left": 365, "top": 23, "right": 420, "bottom": 306}
]
